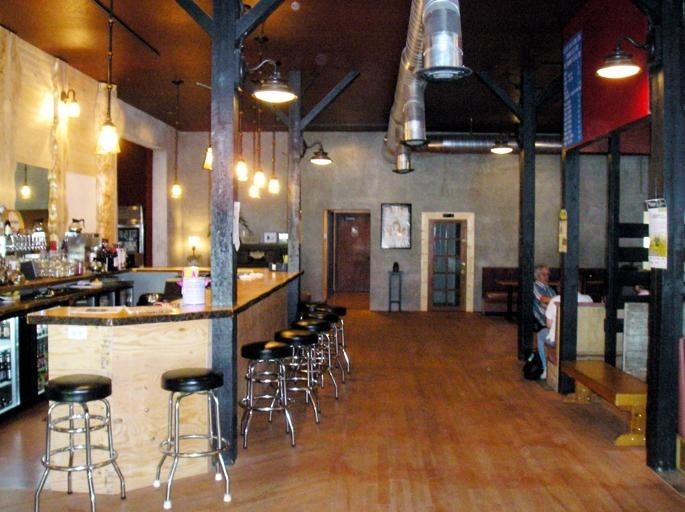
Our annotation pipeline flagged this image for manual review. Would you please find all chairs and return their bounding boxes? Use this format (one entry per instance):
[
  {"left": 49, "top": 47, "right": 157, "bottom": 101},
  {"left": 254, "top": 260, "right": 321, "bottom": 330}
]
[{"left": 674, "top": 336, "right": 685, "bottom": 473}]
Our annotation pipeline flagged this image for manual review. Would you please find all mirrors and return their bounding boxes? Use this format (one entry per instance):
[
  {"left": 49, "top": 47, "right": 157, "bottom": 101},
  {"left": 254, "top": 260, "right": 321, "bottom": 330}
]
[{"left": 14, "top": 161, "right": 58, "bottom": 253}]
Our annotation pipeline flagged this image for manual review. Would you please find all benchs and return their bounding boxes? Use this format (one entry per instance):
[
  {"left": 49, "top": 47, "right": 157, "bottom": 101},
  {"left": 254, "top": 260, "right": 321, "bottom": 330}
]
[
  {"left": 538, "top": 301, "right": 624, "bottom": 388},
  {"left": 560, "top": 354, "right": 649, "bottom": 445},
  {"left": 621, "top": 302, "right": 685, "bottom": 383},
  {"left": 481, "top": 266, "right": 604, "bottom": 318}
]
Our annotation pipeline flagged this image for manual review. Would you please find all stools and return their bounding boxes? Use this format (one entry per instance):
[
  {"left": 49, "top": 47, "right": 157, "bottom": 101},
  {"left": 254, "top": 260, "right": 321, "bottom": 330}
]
[
  {"left": 240, "top": 297, "right": 351, "bottom": 447},
  {"left": 153, "top": 367, "right": 232, "bottom": 508},
  {"left": 35, "top": 373, "right": 126, "bottom": 511}
]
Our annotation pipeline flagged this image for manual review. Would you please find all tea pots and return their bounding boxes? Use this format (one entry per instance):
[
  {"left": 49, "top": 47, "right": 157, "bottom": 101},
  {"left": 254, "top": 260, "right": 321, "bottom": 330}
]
[
  {"left": 30, "top": 218, "right": 45, "bottom": 232},
  {"left": 67, "top": 218, "right": 86, "bottom": 233}
]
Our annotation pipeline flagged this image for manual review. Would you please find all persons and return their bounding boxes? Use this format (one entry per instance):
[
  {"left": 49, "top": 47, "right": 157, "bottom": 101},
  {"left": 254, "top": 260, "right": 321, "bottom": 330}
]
[
  {"left": 536, "top": 273, "right": 595, "bottom": 382},
  {"left": 532, "top": 264, "right": 557, "bottom": 326},
  {"left": 620, "top": 264, "right": 650, "bottom": 296}
]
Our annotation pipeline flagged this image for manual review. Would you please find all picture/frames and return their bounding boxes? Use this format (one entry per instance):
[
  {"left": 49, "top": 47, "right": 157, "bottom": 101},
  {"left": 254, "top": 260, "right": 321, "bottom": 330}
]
[{"left": 381, "top": 203, "right": 411, "bottom": 249}]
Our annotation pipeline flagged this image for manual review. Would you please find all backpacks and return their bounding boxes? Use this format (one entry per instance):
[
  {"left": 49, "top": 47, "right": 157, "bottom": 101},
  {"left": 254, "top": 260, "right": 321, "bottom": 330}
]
[{"left": 523, "top": 352, "right": 544, "bottom": 381}]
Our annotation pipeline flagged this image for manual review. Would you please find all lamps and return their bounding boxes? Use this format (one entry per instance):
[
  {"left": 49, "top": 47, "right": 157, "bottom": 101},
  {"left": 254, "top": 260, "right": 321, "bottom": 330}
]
[
  {"left": 169, "top": 80, "right": 183, "bottom": 201},
  {"left": 20, "top": 161, "right": 32, "bottom": 200},
  {"left": 95, "top": 14, "right": 121, "bottom": 154},
  {"left": 301, "top": 137, "right": 333, "bottom": 165},
  {"left": 186, "top": 234, "right": 202, "bottom": 267},
  {"left": 490, "top": 132, "right": 524, "bottom": 156},
  {"left": 240, "top": 57, "right": 299, "bottom": 105},
  {"left": 198, "top": 82, "right": 281, "bottom": 200},
  {"left": 597, "top": 33, "right": 661, "bottom": 82},
  {"left": 59, "top": 88, "right": 82, "bottom": 122}
]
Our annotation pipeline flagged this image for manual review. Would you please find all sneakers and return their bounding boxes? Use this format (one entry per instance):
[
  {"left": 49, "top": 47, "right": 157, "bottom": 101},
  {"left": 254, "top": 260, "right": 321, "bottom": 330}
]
[{"left": 540, "top": 369, "right": 547, "bottom": 380}]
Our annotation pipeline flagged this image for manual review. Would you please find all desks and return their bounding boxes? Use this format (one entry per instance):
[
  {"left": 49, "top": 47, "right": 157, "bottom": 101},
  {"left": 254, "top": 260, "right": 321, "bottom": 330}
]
[{"left": 388, "top": 271, "right": 403, "bottom": 312}]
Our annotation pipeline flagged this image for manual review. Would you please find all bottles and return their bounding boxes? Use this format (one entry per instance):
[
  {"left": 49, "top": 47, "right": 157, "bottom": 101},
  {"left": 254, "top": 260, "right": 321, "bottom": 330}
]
[
  {"left": 37, "top": 325, "right": 49, "bottom": 392},
  {"left": 0, "top": 321, "right": 11, "bottom": 410}
]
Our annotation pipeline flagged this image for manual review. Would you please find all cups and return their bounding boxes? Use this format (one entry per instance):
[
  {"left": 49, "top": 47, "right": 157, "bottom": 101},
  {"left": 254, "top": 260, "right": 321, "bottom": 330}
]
[
  {"left": 183, "top": 267, "right": 198, "bottom": 278},
  {"left": 182, "top": 278, "right": 205, "bottom": 305},
  {"left": 37, "top": 256, "right": 85, "bottom": 279}
]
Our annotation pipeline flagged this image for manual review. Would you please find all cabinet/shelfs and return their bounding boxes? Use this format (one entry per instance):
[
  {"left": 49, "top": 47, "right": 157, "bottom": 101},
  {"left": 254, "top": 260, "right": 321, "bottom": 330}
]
[
  {"left": 0, "top": 244, "right": 132, "bottom": 293},
  {"left": 0, "top": 310, "right": 33, "bottom": 420},
  {"left": 32, "top": 280, "right": 134, "bottom": 401},
  {"left": 236, "top": 243, "right": 300, "bottom": 269}
]
